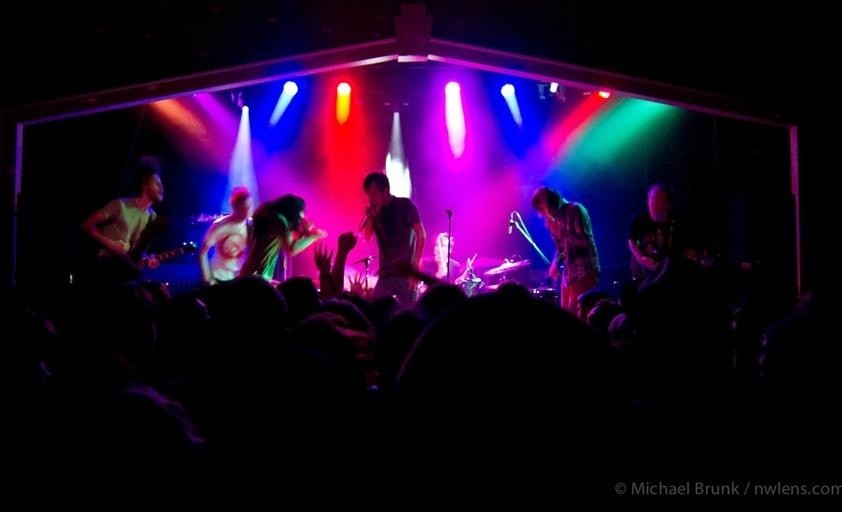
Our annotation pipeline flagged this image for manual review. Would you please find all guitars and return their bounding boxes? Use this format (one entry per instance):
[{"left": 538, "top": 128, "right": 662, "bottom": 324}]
[{"left": 135, "top": 242, "right": 197, "bottom": 267}]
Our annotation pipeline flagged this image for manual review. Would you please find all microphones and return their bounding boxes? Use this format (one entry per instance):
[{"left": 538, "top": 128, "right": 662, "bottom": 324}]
[
  {"left": 441, "top": 205, "right": 454, "bottom": 219},
  {"left": 506, "top": 214, "right": 517, "bottom": 233},
  {"left": 361, "top": 205, "right": 376, "bottom": 230}
]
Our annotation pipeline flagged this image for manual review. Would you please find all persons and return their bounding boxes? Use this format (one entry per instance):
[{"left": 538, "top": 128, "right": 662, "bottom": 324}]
[
  {"left": 198, "top": 186, "right": 256, "bottom": 290},
  {"left": 0, "top": 233, "right": 842, "bottom": 511},
  {"left": 80, "top": 171, "right": 167, "bottom": 283},
  {"left": 624, "top": 183, "right": 713, "bottom": 286},
  {"left": 532, "top": 185, "right": 600, "bottom": 318},
  {"left": 364, "top": 172, "right": 429, "bottom": 302},
  {"left": 238, "top": 194, "right": 328, "bottom": 287}
]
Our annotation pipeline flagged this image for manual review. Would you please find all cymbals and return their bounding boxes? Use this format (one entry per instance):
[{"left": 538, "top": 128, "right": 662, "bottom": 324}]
[
  {"left": 350, "top": 255, "right": 378, "bottom": 266},
  {"left": 484, "top": 260, "right": 528, "bottom": 276}
]
